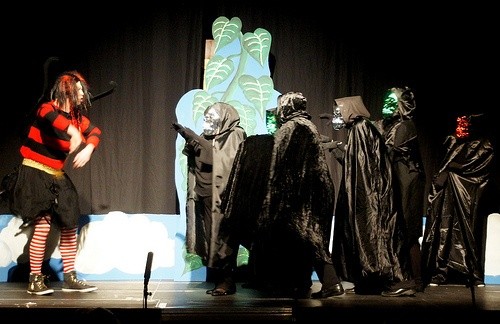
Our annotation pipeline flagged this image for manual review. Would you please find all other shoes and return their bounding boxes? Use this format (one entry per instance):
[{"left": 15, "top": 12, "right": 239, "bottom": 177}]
[
  {"left": 206, "top": 284, "right": 236, "bottom": 296},
  {"left": 431, "top": 274, "right": 445, "bottom": 284},
  {"left": 381, "top": 288, "right": 416, "bottom": 297},
  {"left": 474, "top": 274, "right": 485, "bottom": 286}
]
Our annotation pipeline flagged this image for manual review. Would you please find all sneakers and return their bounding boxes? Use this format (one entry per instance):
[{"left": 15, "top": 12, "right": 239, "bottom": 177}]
[
  {"left": 61, "top": 271, "right": 97, "bottom": 293},
  {"left": 27, "top": 273, "right": 54, "bottom": 295}
]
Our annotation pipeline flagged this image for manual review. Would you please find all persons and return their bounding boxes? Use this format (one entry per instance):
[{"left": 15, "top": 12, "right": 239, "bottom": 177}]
[
  {"left": 370, "top": 87, "right": 415, "bottom": 226},
  {"left": 425, "top": 115, "right": 494, "bottom": 286},
  {"left": 13, "top": 70, "right": 101, "bottom": 296},
  {"left": 324, "top": 95, "right": 415, "bottom": 296},
  {"left": 170, "top": 101, "right": 246, "bottom": 296},
  {"left": 260, "top": 91, "right": 344, "bottom": 297}
]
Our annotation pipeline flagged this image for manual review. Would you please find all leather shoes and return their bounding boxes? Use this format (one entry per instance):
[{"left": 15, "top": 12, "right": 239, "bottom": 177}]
[
  {"left": 284, "top": 287, "right": 310, "bottom": 299},
  {"left": 311, "top": 283, "right": 344, "bottom": 298}
]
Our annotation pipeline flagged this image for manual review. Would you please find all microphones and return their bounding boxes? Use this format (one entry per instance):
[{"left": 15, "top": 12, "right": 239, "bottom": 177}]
[{"left": 143, "top": 252, "right": 153, "bottom": 297}]
